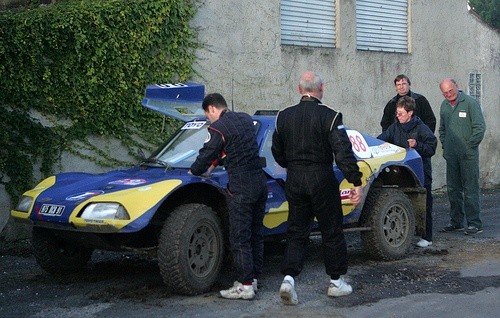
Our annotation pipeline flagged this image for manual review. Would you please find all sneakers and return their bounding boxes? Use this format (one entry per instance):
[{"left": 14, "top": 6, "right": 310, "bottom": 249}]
[
  {"left": 252, "top": 278, "right": 258, "bottom": 294},
  {"left": 327, "top": 275, "right": 352, "bottom": 297},
  {"left": 416, "top": 238, "right": 433, "bottom": 247},
  {"left": 279, "top": 274, "right": 299, "bottom": 306},
  {"left": 220, "top": 280, "right": 255, "bottom": 300}
]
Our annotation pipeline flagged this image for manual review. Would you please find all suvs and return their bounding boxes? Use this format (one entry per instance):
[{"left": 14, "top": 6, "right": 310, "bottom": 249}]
[{"left": 10, "top": 82, "right": 429, "bottom": 295}]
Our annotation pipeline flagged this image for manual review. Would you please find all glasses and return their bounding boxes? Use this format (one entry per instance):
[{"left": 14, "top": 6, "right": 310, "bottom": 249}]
[{"left": 395, "top": 111, "right": 409, "bottom": 116}]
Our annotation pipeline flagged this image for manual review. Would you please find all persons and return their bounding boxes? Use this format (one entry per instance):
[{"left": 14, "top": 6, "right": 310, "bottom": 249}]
[
  {"left": 376, "top": 96, "right": 438, "bottom": 248},
  {"left": 439, "top": 79, "right": 486, "bottom": 236},
  {"left": 271, "top": 72, "right": 363, "bottom": 305},
  {"left": 380, "top": 73, "right": 436, "bottom": 136},
  {"left": 187, "top": 93, "right": 268, "bottom": 301}
]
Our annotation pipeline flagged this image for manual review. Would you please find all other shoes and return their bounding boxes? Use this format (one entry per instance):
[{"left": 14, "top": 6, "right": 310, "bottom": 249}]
[
  {"left": 464, "top": 223, "right": 483, "bottom": 235},
  {"left": 442, "top": 221, "right": 465, "bottom": 233}
]
